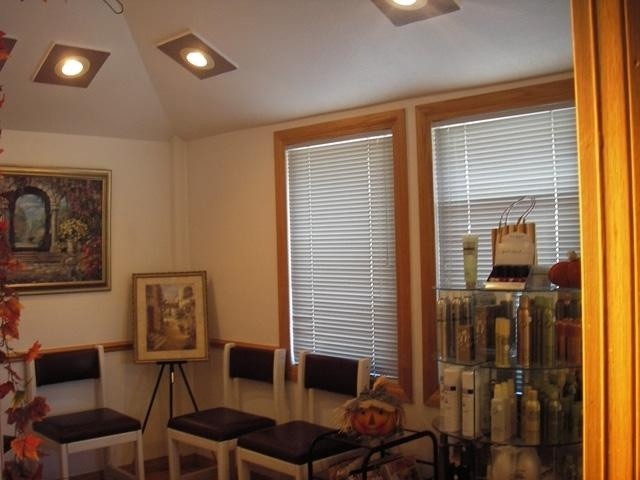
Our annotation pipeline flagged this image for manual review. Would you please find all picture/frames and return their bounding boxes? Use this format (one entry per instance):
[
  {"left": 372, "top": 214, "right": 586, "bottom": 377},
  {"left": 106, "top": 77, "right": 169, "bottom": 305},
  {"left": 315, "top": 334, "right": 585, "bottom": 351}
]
[
  {"left": 0, "top": 164, "right": 111, "bottom": 293},
  {"left": 132, "top": 270, "right": 210, "bottom": 363}
]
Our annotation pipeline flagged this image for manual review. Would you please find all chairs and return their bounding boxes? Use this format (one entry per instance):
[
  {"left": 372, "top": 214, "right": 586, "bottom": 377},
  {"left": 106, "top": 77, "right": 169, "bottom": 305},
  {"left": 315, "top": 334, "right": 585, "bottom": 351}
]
[
  {"left": 25, "top": 345, "right": 146, "bottom": 480},
  {"left": 236, "top": 350, "right": 372, "bottom": 479},
  {"left": 167, "top": 343, "right": 286, "bottom": 479}
]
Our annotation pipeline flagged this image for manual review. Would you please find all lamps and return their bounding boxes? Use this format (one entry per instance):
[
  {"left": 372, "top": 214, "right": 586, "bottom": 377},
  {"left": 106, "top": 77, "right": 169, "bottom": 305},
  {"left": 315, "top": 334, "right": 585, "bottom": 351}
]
[
  {"left": 155, "top": 28, "right": 239, "bottom": 82},
  {"left": 32, "top": 42, "right": 111, "bottom": 90},
  {"left": 371, "top": 0, "right": 464, "bottom": 27}
]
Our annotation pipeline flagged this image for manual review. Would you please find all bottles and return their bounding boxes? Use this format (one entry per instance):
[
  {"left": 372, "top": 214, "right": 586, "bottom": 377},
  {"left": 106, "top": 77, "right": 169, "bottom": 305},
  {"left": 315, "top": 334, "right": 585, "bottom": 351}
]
[{"left": 434, "top": 291, "right": 582, "bottom": 480}]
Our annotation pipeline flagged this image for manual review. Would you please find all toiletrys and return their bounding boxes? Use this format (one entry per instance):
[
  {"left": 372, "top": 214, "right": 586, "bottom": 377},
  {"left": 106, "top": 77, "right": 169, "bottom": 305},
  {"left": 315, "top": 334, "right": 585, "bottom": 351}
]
[
  {"left": 463, "top": 236, "right": 476, "bottom": 287},
  {"left": 446, "top": 445, "right": 578, "bottom": 479},
  {"left": 435, "top": 294, "right": 582, "bottom": 366},
  {"left": 440, "top": 368, "right": 583, "bottom": 441}
]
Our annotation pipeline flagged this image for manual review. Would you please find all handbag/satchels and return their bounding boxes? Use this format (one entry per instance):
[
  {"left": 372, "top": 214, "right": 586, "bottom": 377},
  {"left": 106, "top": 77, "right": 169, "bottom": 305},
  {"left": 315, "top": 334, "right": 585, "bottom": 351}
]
[{"left": 490, "top": 222, "right": 537, "bottom": 267}]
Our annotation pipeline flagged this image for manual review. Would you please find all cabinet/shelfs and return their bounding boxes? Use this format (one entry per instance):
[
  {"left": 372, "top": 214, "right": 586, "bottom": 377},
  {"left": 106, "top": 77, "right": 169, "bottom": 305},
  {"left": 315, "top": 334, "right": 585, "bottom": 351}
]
[{"left": 434, "top": 277, "right": 584, "bottom": 480}]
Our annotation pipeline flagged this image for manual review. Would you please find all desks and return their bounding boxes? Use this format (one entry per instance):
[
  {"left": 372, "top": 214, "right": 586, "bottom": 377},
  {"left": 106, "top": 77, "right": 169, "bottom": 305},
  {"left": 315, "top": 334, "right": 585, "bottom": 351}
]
[{"left": 308, "top": 425, "right": 440, "bottom": 479}]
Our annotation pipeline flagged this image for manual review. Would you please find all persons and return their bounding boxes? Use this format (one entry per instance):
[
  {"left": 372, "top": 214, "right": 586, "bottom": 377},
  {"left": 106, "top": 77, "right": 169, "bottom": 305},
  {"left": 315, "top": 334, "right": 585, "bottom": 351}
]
[{"left": 334, "top": 376, "right": 404, "bottom": 480}]
